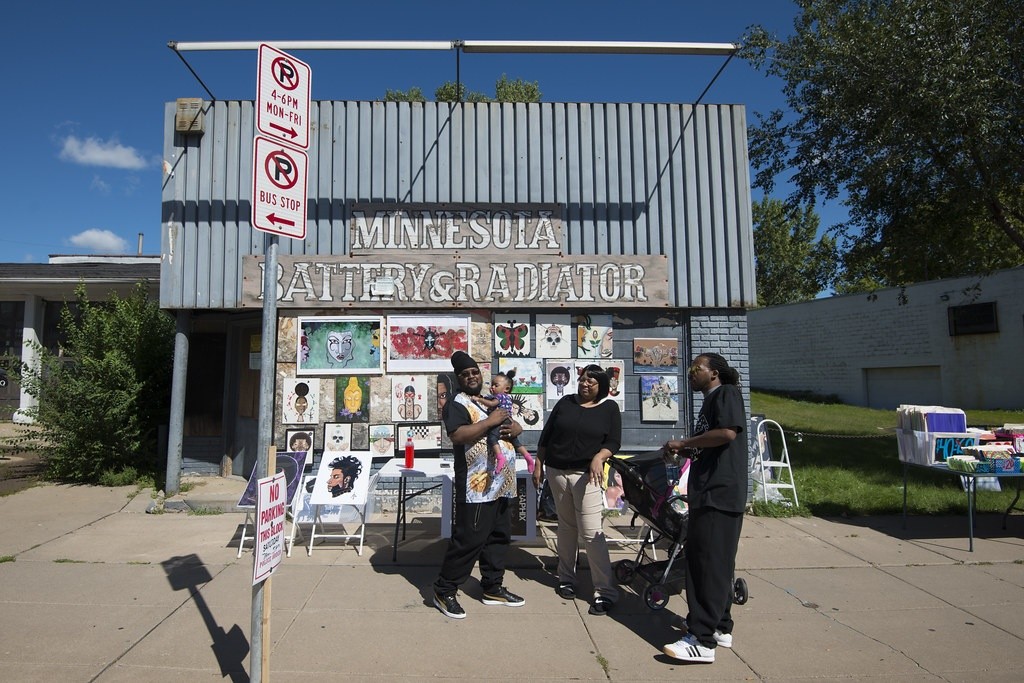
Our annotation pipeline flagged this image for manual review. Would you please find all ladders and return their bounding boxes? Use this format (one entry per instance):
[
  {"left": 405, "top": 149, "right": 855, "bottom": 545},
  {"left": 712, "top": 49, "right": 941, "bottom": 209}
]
[{"left": 753, "top": 419, "right": 800, "bottom": 509}]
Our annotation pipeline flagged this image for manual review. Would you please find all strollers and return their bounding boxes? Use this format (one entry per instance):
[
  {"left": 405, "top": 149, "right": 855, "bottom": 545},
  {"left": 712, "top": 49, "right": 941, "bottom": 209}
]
[{"left": 605, "top": 442, "right": 748, "bottom": 611}]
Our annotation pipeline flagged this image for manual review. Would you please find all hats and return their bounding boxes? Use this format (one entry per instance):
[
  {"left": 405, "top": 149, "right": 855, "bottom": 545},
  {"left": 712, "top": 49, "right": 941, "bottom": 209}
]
[{"left": 450, "top": 351, "right": 479, "bottom": 376}]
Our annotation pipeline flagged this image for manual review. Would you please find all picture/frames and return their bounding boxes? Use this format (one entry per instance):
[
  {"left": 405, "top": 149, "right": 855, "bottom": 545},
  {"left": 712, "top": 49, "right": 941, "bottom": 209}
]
[
  {"left": 368, "top": 424, "right": 395, "bottom": 458},
  {"left": 322, "top": 421, "right": 353, "bottom": 451},
  {"left": 397, "top": 421, "right": 444, "bottom": 454},
  {"left": 285, "top": 427, "right": 315, "bottom": 466}
]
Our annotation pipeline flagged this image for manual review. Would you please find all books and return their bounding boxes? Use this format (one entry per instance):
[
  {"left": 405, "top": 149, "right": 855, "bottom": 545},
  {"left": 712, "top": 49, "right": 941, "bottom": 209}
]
[{"left": 896, "top": 404, "right": 1024, "bottom": 474}]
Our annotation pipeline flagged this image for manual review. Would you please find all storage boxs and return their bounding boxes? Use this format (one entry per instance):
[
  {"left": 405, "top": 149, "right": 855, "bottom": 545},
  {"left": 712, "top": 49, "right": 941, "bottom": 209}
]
[
  {"left": 995, "top": 431, "right": 1024, "bottom": 452},
  {"left": 975, "top": 462, "right": 992, "bottom": 473},
  {"left": 893, "top": 426, "right": 980, "bottom": 466},
  {"left": 987, "top": 457, "right": 1020, "bottom": 473}
]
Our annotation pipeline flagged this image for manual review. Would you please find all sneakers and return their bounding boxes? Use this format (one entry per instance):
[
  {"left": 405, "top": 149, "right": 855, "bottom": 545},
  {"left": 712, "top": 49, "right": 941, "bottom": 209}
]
[
  {"left": 555, "top": 579, "right": 576, "bottom": 598},
  {"left": 589, "top": 597, "right": 613, "bottom": 615},
  {"left": 479, "top": 581, "right": 525, "bottom": 606},
  {"left": 686, "top": 627, "right": 733, "bottom": 648},
  {"left": 662, "top": 630, "right": 716, "bottom": 663},
  {"left": 432, "top": 590, "right": 466, "bottom": 619}
]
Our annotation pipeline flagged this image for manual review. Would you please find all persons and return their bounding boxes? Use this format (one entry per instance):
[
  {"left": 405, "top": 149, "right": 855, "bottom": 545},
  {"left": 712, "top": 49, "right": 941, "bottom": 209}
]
[
  {"left": 533, "top": 363, "right": 623, "bottom": 614},
  {"left": 430, "top": 350, "right": 526, "bottom": 619},
  {"left": 663, "top": 353, "right": 748, "bottom": 664},
  {"left": 470, "top": 370, "right": 535, "bottom": 475}
]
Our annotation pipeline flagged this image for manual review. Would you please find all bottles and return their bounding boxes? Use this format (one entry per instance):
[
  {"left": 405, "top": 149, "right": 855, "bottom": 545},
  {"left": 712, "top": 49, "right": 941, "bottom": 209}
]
[
  {"left": 665, "top": 451, "right": 680, "bottom": 486},
  {"left": 405, "top": 437, "right": 414, "bottom": 468}
]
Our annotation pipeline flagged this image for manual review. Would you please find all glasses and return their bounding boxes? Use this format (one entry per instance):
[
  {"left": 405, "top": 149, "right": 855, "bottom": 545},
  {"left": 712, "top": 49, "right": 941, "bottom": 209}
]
[
  {"left": 458, "top": 369, "right": 481, "bottom": 379},
  {"left": 687, "top": 365, "right": 715, "bottom": 376},
  {"left": 577, "top": 377, "right": 599, "bottom": 387}
]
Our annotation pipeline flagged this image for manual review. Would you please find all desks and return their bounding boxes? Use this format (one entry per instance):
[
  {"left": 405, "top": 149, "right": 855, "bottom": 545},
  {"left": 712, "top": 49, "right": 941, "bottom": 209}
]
[
  {"left": 379, "top": 456, "right": 546, "bottom": 568},
  {"left": 900, "top": 460, "right": 1024, "bottom": 552}
]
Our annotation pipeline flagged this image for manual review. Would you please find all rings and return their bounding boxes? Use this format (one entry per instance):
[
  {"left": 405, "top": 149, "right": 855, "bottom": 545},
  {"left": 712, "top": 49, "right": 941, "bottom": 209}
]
[{"left": 508, "top": 433, "right": 511, "bottom": 437}]
